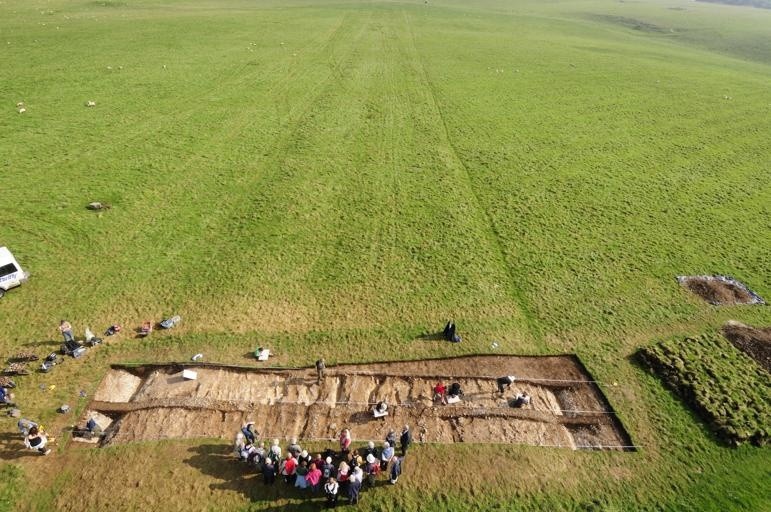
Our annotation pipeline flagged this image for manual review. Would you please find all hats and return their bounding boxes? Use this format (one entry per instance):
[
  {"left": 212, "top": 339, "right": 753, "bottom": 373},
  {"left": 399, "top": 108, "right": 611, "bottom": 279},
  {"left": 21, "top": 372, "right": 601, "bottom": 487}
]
[
  {"left": 508, "top": 375, "right": 515, "bottom": 382},
  {"left": 235, "top": 433, "right": 376, "bottom": 483},
  {"left": 381, "top": 403, "right": 387, "bottom": 411}
]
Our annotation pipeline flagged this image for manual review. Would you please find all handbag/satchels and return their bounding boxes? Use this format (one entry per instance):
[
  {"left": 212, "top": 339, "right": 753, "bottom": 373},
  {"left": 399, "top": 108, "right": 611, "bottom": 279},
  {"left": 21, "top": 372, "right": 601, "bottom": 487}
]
[
  {"left": 107, "top": 325, "right": 121, "bottom": 335},
  {"left": 47, "top": 352, "right": 56, "bottom": 360}
]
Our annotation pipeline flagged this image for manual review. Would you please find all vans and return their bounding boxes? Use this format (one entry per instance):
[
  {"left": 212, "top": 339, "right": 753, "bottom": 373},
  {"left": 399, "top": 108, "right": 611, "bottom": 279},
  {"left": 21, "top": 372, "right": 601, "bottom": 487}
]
[{"left": 0, "top": 246, "right": 25, "bottom": 299}]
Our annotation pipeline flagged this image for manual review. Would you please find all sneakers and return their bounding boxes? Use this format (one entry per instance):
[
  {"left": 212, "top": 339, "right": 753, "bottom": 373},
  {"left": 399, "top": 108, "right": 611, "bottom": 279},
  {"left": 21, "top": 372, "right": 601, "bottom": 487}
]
[{"left": 44, "top": 449, "right": 52, "bottom": 455}]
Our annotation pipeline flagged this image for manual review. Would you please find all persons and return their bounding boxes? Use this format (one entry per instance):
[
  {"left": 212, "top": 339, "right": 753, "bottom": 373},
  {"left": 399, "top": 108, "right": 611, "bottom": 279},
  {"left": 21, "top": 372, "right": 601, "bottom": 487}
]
[
  {"left": 431, "top": 379, "right": 447, "bottom": 406},
  {"left": 316, "top": 359, "right": 324, "bottom": 386},
  {"left": 514, "top": 392, "right": 528, "bottom": 408},
  {"left": 497, "top": 376, "right": 515, "bottom": 393},
  {"left": 24, "top": 427, "right": 51, "bottom": 455},
  {"left": 442, "top": 318, "right": 459, "bottom": 342},
  {"left": 368, "top": 401, "right": 387, "bottom": 421},
  {"left": 59, "top": 319, "right": 74, "bottom": 342},
  {"left": 255, "top": 347, "right": 273, "bottom": 358},
  {"left": 235, "top": 425, "right": 411, "bottom": 508}
]
